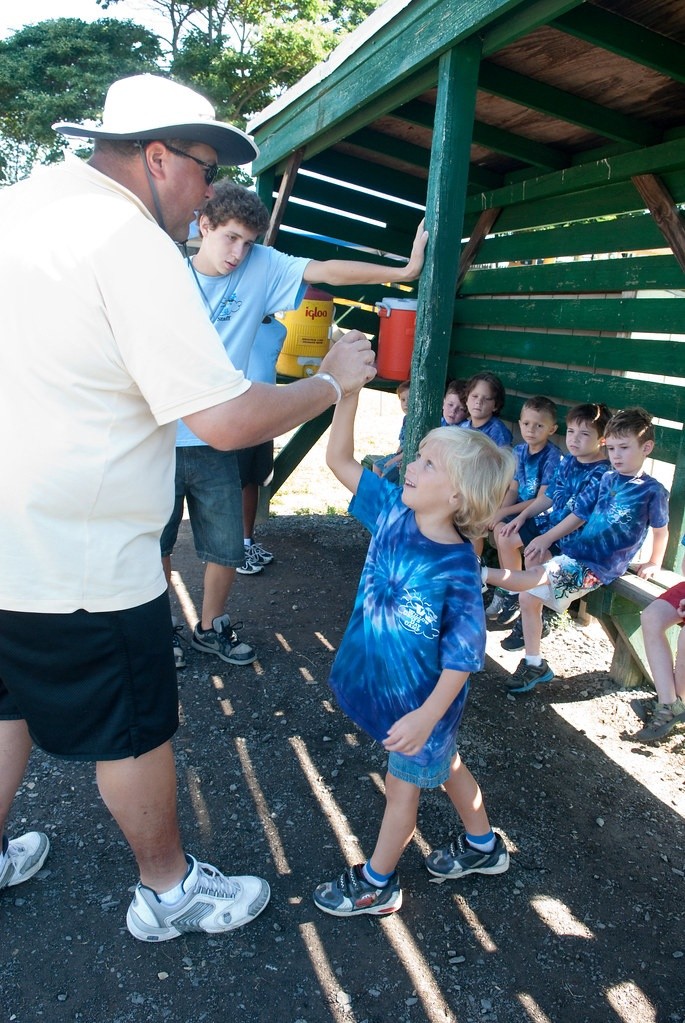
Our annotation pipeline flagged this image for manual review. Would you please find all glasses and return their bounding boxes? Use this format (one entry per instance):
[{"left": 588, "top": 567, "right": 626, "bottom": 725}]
[{"left": 136, "top": 140, "right": 220, "bottom": 185}]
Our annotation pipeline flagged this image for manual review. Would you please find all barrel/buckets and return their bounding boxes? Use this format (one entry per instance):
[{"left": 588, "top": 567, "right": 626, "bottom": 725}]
[
  {"left": 375, "top": 296, "right": 418, "bottom": 382},
  {"left": 274, "top": 285, "right": 334, "bottom": 379}
]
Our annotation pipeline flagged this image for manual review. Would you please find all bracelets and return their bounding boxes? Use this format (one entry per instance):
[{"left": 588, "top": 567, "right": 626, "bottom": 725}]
[{"left": 312, "top": 372, "right": 343, "bottom": 404}]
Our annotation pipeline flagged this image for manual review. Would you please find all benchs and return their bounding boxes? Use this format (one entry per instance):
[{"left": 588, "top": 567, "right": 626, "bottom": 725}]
[{"left": 361, "top": 455, "right": 685, "bottom": 689}]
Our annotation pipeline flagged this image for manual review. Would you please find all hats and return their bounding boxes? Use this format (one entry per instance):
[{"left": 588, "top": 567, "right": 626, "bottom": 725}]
[{"left": 51, "top": 74, "right": 259, "bottom": 166}]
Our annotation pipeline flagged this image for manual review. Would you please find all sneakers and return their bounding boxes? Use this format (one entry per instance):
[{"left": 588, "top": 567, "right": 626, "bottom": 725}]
[
  {"left": 189, "top": 613, "right": 258, "bottom": 666},
  {"left": 168, "top": 625, "right": 187, "bottom": 668},
  {"left": 0, "top": 831, "right": 50, "bottom": 891},
  {"left": 500, "top": 613, "right": 551, "bottom": 652},
  {"left": 126, "top": 853, "right": 272, "bottom": 943},
  {"left": 501, "top": 658, "right": 555, "bottom": 694},
  {"left": 496, "top": 593, "right": 522, "bottom": 626},
  {"left": 636, "top": 697, "right": 685, "bottom": 741},
  {"left": 235, "top": 546, "right": 265, "bottom": 576},
  {"left": 484, "top": 587, "right": 509, "bottom": 621},
  {"left": 311, "top": 862, "right": 404, "bottom": 917},
  {"left": 424, "top": 832, "right": 510, "bottom": 878},
  {"left": 474, "top": 554, "right": 489, "bottom": 594},
  {"left": 242, "top": 542, "right": 274, "bottom": 565}
]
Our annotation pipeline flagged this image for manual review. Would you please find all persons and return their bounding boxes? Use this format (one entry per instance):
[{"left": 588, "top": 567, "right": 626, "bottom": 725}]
[
  {"left": 313, "top": 391, "right": 520, "bottom": 916},
  {"left": 503, "top": 406, "right": 669, "bottom": 692},
  {"left": 0, "top": 71, "right": 376, "bottom": 942},
  {"left": 635, "top": 535, "right": 685, "bottom": 741},
  {"left": 160, "top": 181, "right": 428, "bottom": 667},
  {"left": 373, "top": 372, "right": 612, "bottom": 652}
]
